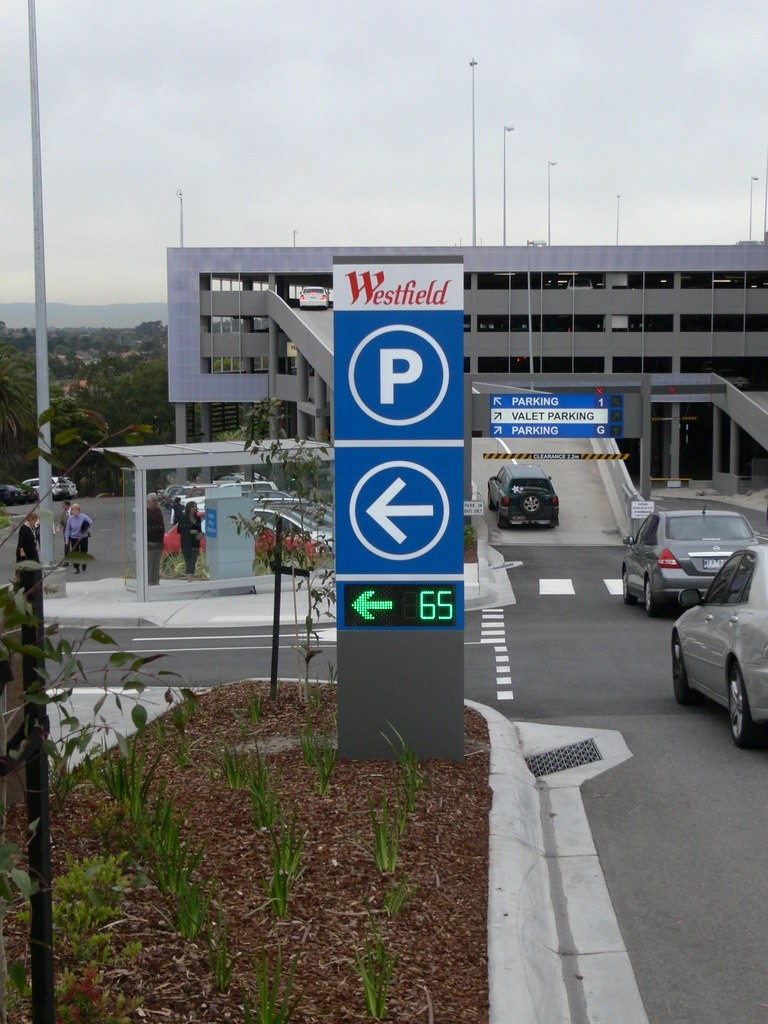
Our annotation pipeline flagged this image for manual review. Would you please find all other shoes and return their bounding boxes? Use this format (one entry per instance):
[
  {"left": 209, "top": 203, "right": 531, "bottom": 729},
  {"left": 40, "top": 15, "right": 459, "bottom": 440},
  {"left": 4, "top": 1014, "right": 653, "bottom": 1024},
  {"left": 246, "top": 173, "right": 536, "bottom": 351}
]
[
  {"left": 82, "top": 566, "right": 86, "bottom": 571},
  {"left": 62, "top": 562, "right": 69, "bottom": 566},
  {"left": 74, "top": 570, "right": 80, "bottom": 574}
]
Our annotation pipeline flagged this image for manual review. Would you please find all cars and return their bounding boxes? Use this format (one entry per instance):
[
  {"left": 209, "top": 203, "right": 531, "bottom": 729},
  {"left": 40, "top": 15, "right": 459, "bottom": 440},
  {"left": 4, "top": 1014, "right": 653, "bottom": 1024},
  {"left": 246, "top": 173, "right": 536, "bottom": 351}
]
[
  {"left": 671, "top": 542, "right": 768, "bottom": 749},
  {"left": 0, "top": 484, "right": 38, "bottom": 506},
  {"left": 702, "top": 361, "right": 751, "bottom": 391},
  {"left": 211, "top": 471, "right": 269, "bottom": 484},
  {"left": 299, "top": 286, "right": 330, "bottom": 311},
  {"left": 620, "top": 509, "right": 760, "bottom": 617},
  {"left": 160, "top": 482, "right": 333, "bottom": 577}
]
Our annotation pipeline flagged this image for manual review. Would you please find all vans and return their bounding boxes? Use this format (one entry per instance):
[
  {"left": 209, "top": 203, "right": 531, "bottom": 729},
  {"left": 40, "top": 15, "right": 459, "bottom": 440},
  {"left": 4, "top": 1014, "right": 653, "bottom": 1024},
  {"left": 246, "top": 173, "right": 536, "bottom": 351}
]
[{"left": 567, "top": 277, "right": 593, "bottom": 290}]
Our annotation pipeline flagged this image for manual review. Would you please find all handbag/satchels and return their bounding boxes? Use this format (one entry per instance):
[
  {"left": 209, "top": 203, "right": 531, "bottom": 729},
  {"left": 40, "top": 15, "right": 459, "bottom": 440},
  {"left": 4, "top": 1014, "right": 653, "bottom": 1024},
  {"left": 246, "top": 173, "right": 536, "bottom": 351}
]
[{"left": 81, "top": 521, "right": 91, "bottom": 537}]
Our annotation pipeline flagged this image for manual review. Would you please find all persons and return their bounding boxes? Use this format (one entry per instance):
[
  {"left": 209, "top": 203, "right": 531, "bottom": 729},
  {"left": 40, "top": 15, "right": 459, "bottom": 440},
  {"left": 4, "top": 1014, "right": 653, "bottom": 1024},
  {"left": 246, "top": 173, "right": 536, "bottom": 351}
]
[
  {"left": 32, "top": 512, "right": 57, "bottom": 562},
  {"left": 14, "top": 512, "right": 41, "bottom": 565},
  {"left": 56, "top": 499, "right": 73, "bottom": 567},
  {"left": 142, "top": 493, "right": 165, "bottom": 586},
  {"left": 177, "top": 501, "right": 201, "bottom": 582},
  {"left": 64, "top": 503, "right": 93, "bottom": 574},
  {"left": 170, "top": 496, "right": 186, "bottom": 525}
]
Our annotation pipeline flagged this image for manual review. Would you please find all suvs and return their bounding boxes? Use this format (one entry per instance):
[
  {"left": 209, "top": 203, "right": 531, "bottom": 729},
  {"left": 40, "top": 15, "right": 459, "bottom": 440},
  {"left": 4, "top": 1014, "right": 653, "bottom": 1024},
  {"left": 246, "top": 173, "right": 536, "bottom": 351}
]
[
  {"left": 64, "top": 477, "right": 79, "bottom": 500},
  {"left": 487, "top": 463, "right": 559, "bottom": 529},
  {"left": 21, "top": 476, "right": 70, "bottom": 501}
]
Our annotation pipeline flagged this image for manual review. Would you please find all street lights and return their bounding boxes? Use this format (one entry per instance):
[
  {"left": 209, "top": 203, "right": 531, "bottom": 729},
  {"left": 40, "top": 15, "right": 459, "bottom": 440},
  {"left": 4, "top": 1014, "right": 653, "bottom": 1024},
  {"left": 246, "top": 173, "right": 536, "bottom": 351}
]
[
  {"left": 749, "top": 177, "right": 758, "bottom": 240},
  {"left": 293, "top": 229, "right": 299, "bottom": 247},
  {"left": 502, "top": 125, "right": 515, "bottom": 246},
  {"left": 175, "top": 188, "right": 184, "bottom": 248},
  {"left": 546, "top": 161, "right": 557, "bottom": 247},
  {"left": 526, "top": 240, "right": 547, "bottom": 390},
  {"left": 615, "top": 196, "right": 623, "bottom": 246},
  {"left": 469, "top": 57, "right": 477, "bottom": 246}
]
